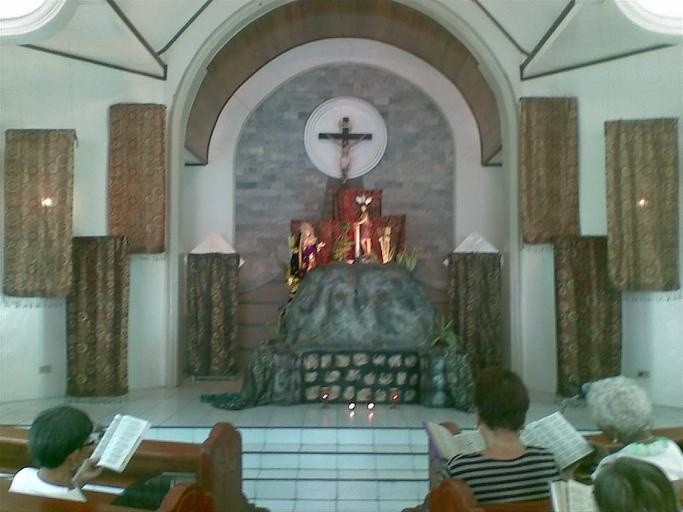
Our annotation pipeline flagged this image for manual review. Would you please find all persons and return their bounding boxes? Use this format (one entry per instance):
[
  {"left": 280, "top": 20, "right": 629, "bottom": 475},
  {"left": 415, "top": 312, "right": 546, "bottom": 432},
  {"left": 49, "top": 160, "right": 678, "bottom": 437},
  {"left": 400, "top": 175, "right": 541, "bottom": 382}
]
[
  {"left": 593, "top": 457, "right": 676, "bottom": 511},
  {"left": 583, "top": 374, "right": 682, "bottom": 484},
  {"left": 436, "top": 369, "right": 561, "bottom": 505},
  {"left": 6, "top": 404, "right": 104, "bottom": 502},
  {"left": 297, "top": 224, "right": 325, "bottom": 272},
  {"left": 326, "top": 133, "right": 367, "bottom": 186},
  {"left": 351, "top": 204, "right": 372, "bottom": 259}
]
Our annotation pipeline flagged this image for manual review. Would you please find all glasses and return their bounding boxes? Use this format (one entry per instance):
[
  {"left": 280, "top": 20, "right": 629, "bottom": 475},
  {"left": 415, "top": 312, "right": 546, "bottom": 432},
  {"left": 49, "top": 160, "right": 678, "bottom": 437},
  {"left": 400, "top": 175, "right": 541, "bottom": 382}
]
[{"left": 77, "top": 438, "right": 94, "bottom": 450}]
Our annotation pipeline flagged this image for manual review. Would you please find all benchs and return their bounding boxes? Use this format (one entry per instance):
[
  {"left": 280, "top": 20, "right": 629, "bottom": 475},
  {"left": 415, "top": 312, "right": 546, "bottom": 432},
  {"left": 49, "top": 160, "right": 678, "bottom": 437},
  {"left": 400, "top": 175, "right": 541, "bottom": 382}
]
[
  {"left": 428, "top": 478, "right": 683, "bottom": 512},
  {"left": 1, "top": 421, "right": 270, "bottom": 511},
  {"left": 429, "top": 421, "right": 683, "bottom": 489},
  {"left": 0, "top": 477, "right": 220, "bottom": 511}
]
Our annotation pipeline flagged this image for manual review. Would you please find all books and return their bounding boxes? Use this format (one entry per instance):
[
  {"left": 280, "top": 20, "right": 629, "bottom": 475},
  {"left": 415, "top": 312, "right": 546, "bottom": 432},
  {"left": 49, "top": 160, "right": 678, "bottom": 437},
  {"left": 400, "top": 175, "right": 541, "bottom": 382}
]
[
  {"left": 420, "top": 418, "right": 485, "bottom": 461},
  {"left": 90, "top": 415, "right": 151, "bottom": 474},
  {"left": 547, "top": 479, "right": 598, "bottom": 512},
  {"left": 520, "top": 410, "right": 595, "bottom": 474}
]
